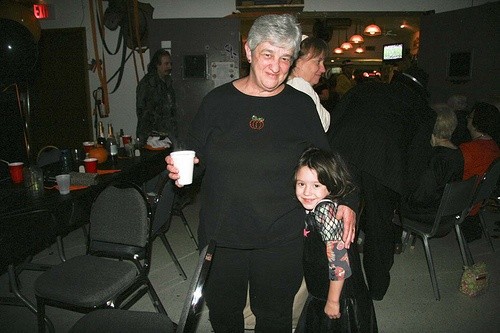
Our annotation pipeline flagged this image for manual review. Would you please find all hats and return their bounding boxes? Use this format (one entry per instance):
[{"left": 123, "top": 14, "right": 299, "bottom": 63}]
[{"left": 395, "top": 67, "right": 433, "bottom": 98}]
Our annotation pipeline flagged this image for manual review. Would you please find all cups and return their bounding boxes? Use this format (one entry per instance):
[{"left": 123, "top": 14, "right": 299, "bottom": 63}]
[
  {"left": 122, "top": 136, "right": 134, "bottom": 144},
  {"left": 23, "top": 164, "right": 43, "bottom": 194},
  {"left": 56, "top": 174, "right": 70, "bottom": 194},
  {"left": 170, "top": 150, "right": 196, "bottom": 185},
  {"left": 84, "top": 158, "right": 98, "bottom": 173},
  {"left": 83, "top": 141, "right": 95, "bottom": 158},
  {"left": 9, "top": 162, "right": 25, "bottom": 183}
]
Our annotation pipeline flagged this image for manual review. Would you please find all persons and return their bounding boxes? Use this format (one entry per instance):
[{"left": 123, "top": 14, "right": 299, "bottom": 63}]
[
  {"left": 293, "top": 146, "right": 378, "bottom": 333},
  {"left": 136, "top": 49, "right": 179, "bottom": 233},
  {"left": 165, "top": 14, "right": 361, "bottom": 333},
  {"left": 284, "top": 33, "right": 330, "bottom": 134},
  {"left": 312, "top": 58, "right": 500, "bottom": 254}
]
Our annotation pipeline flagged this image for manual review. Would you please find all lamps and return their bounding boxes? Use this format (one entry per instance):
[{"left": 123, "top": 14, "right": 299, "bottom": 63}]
[
  {"left": 350, "top": 24, "right": 364, "bottom": 43},
  {"left": 334, "top": 30, "right": 342, "bottom": 53},
  {"left": 341, "top": 32, "right": 353, "bottom": 50},
  {"left": 363, "top": 16, "right": 381, "bottom": 36},
  {"left": 356, "top": 44, "right": 363, "bottom": 53}
]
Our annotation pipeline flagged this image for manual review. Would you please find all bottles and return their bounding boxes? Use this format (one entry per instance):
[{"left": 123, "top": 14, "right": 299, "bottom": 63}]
[
  {"left": 60, "top": 149, "right": 81, "bottom": 173},
  {"left": 97, "top": 121, "right": 125, "bottom": 160}
]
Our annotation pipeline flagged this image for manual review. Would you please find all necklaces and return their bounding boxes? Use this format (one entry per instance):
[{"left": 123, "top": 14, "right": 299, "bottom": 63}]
[
  {"left": 436, "top": 140, "right": 449, "bottom": 144},
  {"left": 476, "top": 135, "right": 484, "bottom": 139}
]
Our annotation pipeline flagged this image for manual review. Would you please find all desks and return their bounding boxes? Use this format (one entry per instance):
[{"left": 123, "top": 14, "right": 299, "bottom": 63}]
[{"left": 0, "top": 149, "right": 177, "bottom": 333}]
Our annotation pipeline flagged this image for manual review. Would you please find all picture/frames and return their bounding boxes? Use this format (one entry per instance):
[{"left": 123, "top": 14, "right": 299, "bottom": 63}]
[{"left": 445, "top": 48, "right": 475, "bottom": 81}]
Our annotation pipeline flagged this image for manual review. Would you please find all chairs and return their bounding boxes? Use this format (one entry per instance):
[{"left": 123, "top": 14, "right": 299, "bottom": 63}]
[
  {"left": 68, "top": 239, "right": 217, "bottom": 333},
  {"left": 403, "top": 175, "right": 477, "bottom": 302},
  {"left": 35, "top": 182, "right": 167, "bottom": 333},
  {"left": 461, "top": 158, "right": 500, "bottom": 266},
  {"left": 148, "top": 178, "right": 201, "bottom": 281}
]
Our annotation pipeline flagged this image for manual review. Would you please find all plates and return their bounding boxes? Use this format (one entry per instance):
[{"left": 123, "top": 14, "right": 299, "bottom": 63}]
[
  {"left": 146, "top": 145, "right": 165, "bottom": 150},
  {"left": 55, "top": 184, "right": 89, "bottom": 190}
]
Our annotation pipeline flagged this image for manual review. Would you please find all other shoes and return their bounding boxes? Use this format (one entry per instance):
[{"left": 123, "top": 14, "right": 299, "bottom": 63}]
[{"left": 394, "top": 242, "right": 403, "bottom": 255}]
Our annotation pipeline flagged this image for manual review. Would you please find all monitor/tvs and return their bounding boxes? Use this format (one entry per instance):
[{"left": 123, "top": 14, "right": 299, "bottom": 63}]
[{"left": 382, "top": 42, "right": 404, "bottom": 61}]
